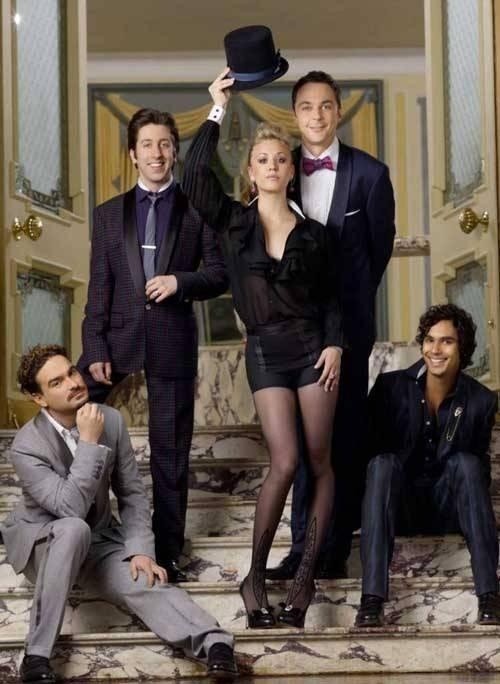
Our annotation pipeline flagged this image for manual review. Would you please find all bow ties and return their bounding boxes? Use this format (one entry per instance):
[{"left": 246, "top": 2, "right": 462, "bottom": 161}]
[{"left": 303, "top": 156, "right": 333, "bottom": 176}]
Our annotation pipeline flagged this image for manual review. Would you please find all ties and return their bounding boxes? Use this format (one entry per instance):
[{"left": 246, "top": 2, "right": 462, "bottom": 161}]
[{"left": 143, "top": 194, "right": 160, "bottom": 281}]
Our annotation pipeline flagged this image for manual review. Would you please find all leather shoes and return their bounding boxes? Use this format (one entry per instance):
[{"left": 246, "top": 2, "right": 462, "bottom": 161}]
[
  {"left": 20, "top": 655, "right": 56, "bottom": 684},
  {"left": 478, "top": 592, "right": 500, "bottom": 625},
  {"left": 240, "top": 551, "right": 348, "bottom": 629},
  {"left": 159, "top": 559, "right": 186, "bottom": 583},
  {"left": 207, "top": 643, "right": 239, "bottom": 678},
  {"left": 357, "top": 595, "right": 384, "bottom": 627}
]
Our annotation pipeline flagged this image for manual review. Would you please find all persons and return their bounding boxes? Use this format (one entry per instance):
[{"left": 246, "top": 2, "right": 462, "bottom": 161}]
[
  {"left": 74, "top": 107, "right": 231, "bottom": 582},
  {"left": 181, "top": 67, "right": 350, "bottom": 629},
  {"left": 244, "top": 70, "right": 395, "bottom": 580},
  {"left": 0, "top": 343, "right": 240, "bottom": 684},
  {"left": 352, "top": 303, "right": 500, "bottom": 624}
]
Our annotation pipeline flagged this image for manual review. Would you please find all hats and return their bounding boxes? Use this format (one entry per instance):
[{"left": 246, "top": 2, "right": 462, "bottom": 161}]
[{"left": 223, "top": 26, "right": 288, "bottom": 90}]
